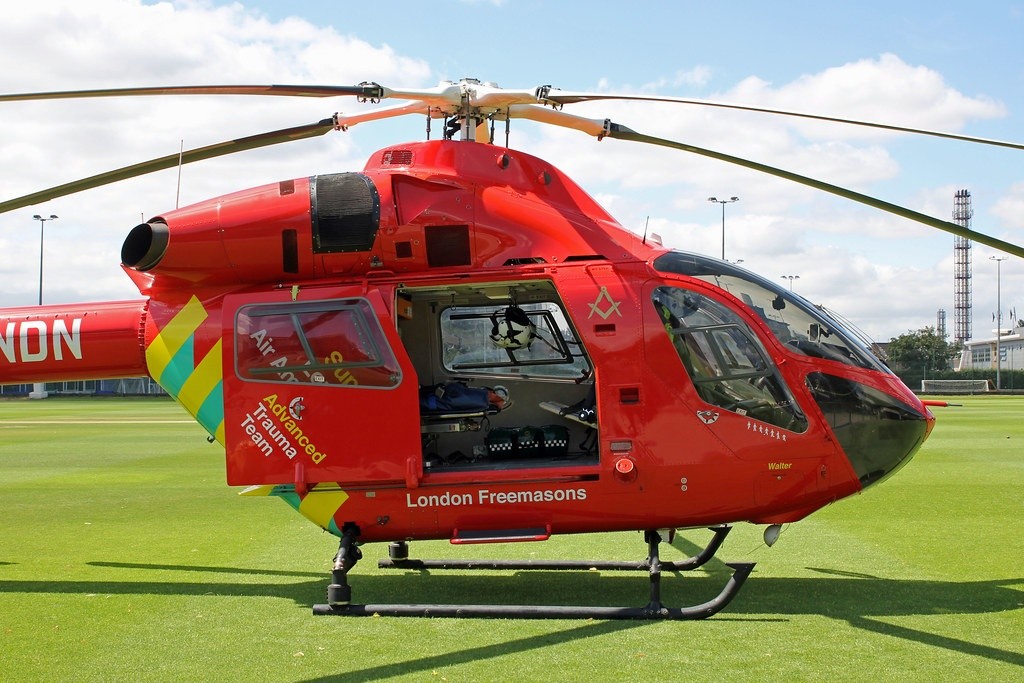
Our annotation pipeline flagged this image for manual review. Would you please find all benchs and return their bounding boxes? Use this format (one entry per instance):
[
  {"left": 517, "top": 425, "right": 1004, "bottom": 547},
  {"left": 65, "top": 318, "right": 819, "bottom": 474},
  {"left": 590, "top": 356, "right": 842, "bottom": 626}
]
[{"left": 538, "top": 401, "right": 597, "bottom": 430}]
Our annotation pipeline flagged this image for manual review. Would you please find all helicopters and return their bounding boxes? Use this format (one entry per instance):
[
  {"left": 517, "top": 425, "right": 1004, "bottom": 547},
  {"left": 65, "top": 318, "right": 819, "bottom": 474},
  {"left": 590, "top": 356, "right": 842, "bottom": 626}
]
[{"left": 0, "top": 77, "right": 1024, "bottom": 621}]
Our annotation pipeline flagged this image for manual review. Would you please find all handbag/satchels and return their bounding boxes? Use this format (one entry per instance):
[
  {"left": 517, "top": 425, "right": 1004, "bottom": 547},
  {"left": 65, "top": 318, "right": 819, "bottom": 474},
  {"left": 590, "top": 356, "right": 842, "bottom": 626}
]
[{"left": 484, "top": 424, "right": 570, "bottom": 463}]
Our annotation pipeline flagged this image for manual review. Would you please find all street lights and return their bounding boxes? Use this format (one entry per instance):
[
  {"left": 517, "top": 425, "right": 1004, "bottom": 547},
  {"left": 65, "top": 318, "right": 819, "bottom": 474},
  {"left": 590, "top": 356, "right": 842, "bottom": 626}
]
[
  {"left": 707, "top": 195, "right": 739, "bottom": 260},
  {"left": 780, "top": 274, "right": 802, "bottom": 292},
  {"left": 32, "top": 214, "right": 59, "bottom": 306},
  {"left": 987, "top": 255, "right": 1010, "bottom": 388}
]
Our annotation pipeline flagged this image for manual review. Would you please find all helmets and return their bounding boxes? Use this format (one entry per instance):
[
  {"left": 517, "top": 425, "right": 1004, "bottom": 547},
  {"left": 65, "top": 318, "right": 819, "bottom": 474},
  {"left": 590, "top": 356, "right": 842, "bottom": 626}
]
[{"left": 489, "top": 307, "right": 537, "bottom": 351}]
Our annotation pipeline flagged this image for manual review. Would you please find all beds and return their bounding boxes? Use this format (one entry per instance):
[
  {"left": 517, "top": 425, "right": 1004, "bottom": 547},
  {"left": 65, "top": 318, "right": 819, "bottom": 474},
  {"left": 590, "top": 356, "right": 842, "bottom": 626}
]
[{"left": 418, "top": 405, "right": 501, "bottom": 468}]
